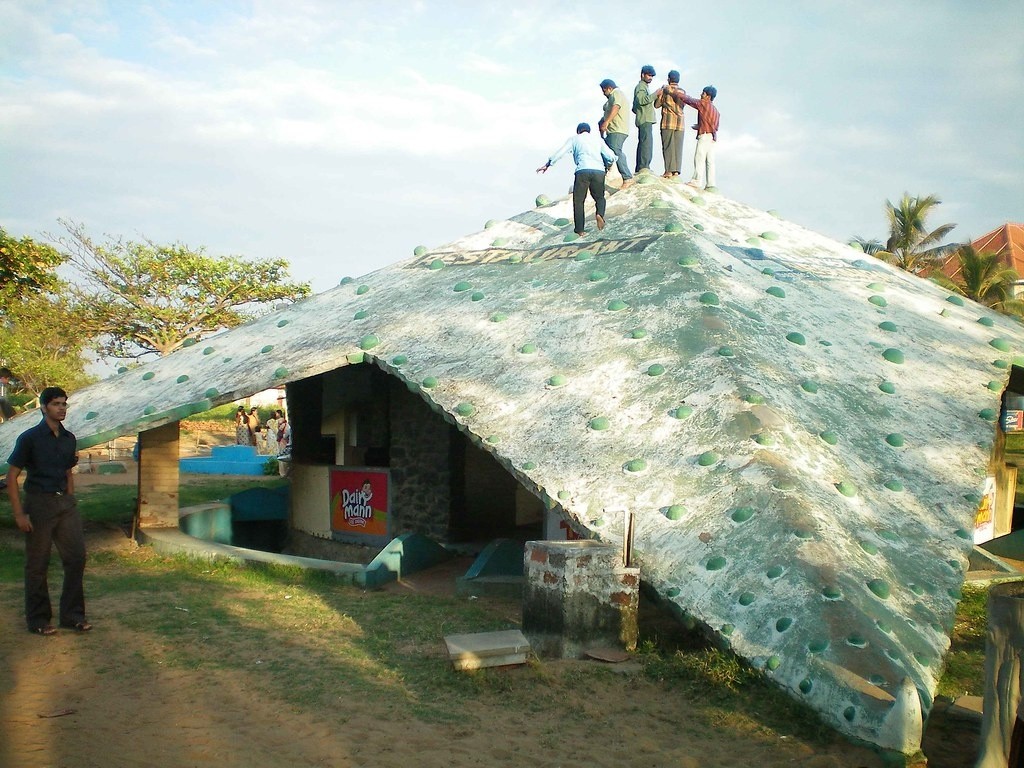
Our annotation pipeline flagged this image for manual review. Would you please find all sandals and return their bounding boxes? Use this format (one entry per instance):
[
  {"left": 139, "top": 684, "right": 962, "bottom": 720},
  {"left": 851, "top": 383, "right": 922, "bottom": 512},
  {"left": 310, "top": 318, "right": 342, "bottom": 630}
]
[
  {"left": 28, "top": 624, "right": 57, "bottom": 635},
  {"left": 60, "top": 620, "right": 93, "bottom": 631}
]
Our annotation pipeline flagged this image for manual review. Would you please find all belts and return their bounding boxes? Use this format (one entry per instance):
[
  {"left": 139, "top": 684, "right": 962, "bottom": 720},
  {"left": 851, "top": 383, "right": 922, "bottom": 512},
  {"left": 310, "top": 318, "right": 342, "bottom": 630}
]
[{"left": 44, "top": 492, "right": 63, "bottom": 496}]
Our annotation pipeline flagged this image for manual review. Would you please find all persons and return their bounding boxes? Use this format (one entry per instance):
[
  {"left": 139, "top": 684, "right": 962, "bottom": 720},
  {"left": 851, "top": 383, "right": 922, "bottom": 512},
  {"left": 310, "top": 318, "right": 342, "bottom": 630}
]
[
  {"left": 599, "top": 78, "right": 634, "bottom": 190},
  {"left": 653, "top": 69, "right": 687, "bottom": 178},
  {"left": 666, "top": 84, "right": 721, "bottom": 191},
  {"left": 233, "top": 404, "right": 292, "bottom": 453},
  {"left": 0, "top": 367, "right": 12, "bottom": 425},
  {"left": 536, "top": 123, "right": 619, "bottom": 236},
  {"left": 631, "top": 64, "right": 663, "bottom": 173},
  {"left": 6, "top": 386, "right": 93, "bottom": 636}
]
